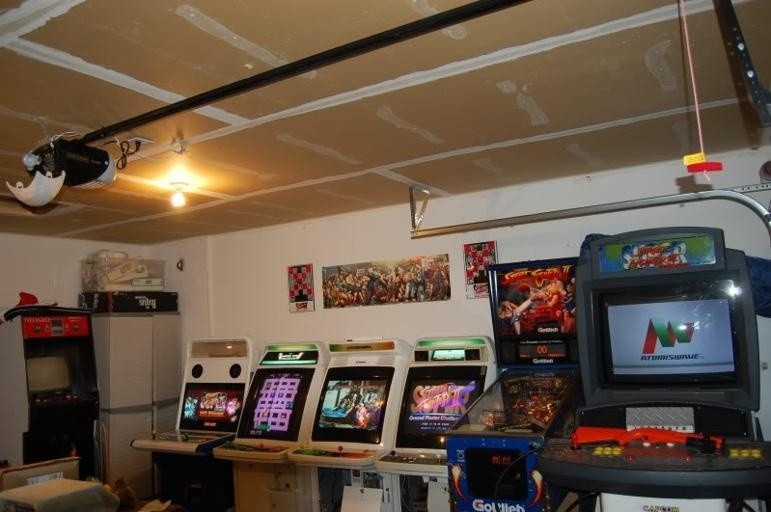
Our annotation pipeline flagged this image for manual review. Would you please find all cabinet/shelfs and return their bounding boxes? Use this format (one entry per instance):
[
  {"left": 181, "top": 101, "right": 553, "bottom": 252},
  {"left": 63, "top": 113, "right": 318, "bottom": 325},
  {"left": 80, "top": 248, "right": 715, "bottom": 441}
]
[
  {"left": 0, "top": 305, "right": 100, "bottom": 467},
  {"left": 537, "top": 226, "right": 770, "bottom": 511},
  {"left": 445, "top": 256, "right": 595, "bottom": 512},
  {"left": 374, "top": 336, "right": 496, "bottom": 511},
  {"left": 288, "top": 338, "right": 413, "bottom": 511},
  {"left": 213, "top": 342, "right": 330, "bottom": 512},
  {"left": 130, "top": 336, "right": 252, "bottom": 512},
  {"left": 77, "top": 291, "right": 181, "bottom": 499}
]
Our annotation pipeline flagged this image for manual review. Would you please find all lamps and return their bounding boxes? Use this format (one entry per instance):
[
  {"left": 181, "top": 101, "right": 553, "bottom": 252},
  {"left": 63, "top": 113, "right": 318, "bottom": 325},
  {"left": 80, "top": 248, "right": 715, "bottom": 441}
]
[{"left": 170, "top": 182, "right": 188, "bottom": 208}]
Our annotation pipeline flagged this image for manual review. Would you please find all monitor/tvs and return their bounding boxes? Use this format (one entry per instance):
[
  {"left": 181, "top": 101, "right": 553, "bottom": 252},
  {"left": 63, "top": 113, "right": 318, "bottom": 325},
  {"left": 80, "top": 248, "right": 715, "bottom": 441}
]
[
  {"left": 311, "top": 367, "right": 396, "bottom": 443},
  {"left": 179, "top": 382, "right": 245, "bottom": 433},
  {"left": 25, "top": 354, "right": 74, "bottom": 398},
  {"left": 598, "top": 281, "right": 743, "bottom": 387},
  {"left": 236, "top": 367, "right": 316, "bottom": 443},
  {"left": 395, "top": 364, "right": 488, "bottom": 449}
]
